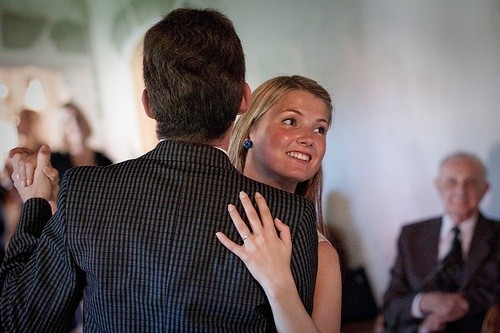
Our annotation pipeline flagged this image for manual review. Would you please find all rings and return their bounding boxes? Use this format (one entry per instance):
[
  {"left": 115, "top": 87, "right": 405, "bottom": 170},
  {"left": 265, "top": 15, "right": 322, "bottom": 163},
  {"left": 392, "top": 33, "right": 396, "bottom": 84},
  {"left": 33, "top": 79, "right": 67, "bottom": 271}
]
[{"left": 242, "top": 231, "right": 251, "bottom": 240}]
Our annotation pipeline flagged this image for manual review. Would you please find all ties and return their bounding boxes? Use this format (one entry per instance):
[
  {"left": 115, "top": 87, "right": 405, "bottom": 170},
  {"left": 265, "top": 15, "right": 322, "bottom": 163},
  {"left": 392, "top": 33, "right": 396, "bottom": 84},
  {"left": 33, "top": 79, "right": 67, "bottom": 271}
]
[{"left": 441, "top": 228, "right": 466, "bottom": 277}]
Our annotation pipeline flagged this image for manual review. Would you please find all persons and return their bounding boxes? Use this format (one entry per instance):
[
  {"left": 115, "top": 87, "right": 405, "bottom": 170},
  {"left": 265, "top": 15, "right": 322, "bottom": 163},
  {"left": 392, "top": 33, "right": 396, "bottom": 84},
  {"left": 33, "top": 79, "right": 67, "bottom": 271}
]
[
  {"left": 1, "top": 107, "right": 47, "bottom": 251},
  {"left": 0, "top": 5, "right": 318, "bottom": 333},
  {"left": 44, "top": 101, "right": 116, "bottom": 188},
  {"left": 10, "top": 74, "right": 342, "bottom": 332},
  {"left": 381, "top": 153, "right": 499, "bottom": 333},
  {"left": 314, "top": 219, "right": 379, "bottom": 332}
]
